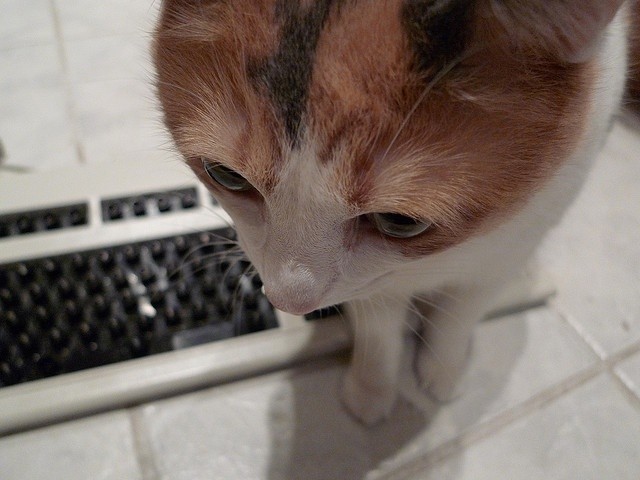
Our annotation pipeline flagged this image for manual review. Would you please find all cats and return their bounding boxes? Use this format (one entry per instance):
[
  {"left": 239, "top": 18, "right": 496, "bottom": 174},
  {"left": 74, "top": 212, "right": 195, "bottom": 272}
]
[{"left": 140, "top": 0, "right": 634, "bottom": 428}]
